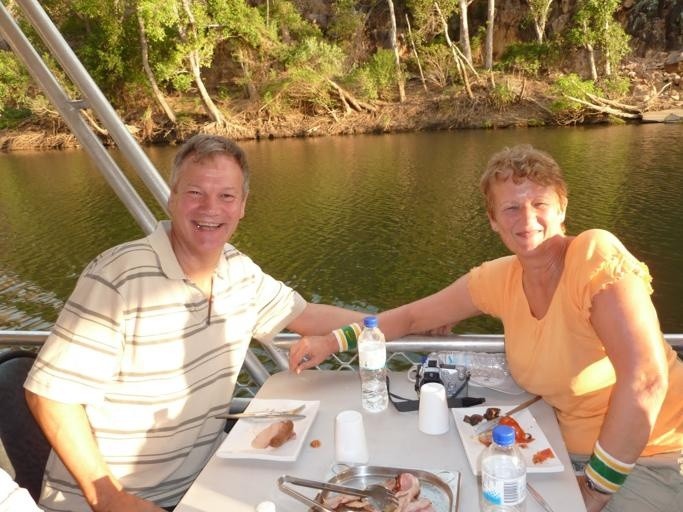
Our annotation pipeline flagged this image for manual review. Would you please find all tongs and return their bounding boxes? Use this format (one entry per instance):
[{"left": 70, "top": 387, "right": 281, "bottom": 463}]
[{"left": 275, "top": 472, "right": 399, "bottom": 512}]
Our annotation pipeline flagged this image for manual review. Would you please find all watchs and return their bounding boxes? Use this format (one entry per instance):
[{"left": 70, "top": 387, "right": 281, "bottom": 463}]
[{"left": 583, "top": 479, "right": 607, "bottom": 496}]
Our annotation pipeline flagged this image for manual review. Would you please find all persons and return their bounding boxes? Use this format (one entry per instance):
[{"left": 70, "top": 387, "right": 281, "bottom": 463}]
[
  {"left": 23, "top": 134, "right": 456, "bottom": 512},
  {"left": 287, "top": 143, "right": 681, "bottom": 510}
]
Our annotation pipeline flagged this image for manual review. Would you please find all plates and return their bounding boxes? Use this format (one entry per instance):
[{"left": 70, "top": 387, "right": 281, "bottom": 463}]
[
  {"left": 216, "top": 397, "right": 323, "bottom": 463},
  {"left": 452, "top": 403, "right": 567, "bottom": 477}
]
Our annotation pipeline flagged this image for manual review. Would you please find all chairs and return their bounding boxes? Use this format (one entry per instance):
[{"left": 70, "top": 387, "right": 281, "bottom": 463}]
[{"left": 0, "top": 348, "right": 52, "bottom": 493}]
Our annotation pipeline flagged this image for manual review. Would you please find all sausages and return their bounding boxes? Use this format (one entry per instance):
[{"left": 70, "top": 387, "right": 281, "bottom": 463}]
[{"left": 269, "top": 421, "right": 294, "bottom": 448}]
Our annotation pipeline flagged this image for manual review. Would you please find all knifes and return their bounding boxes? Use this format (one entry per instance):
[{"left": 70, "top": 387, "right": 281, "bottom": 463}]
[{"left": 211, "top": 410, "right": 307, "bottom": 423}]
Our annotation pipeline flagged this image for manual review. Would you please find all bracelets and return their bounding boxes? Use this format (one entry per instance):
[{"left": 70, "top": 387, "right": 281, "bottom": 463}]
[
  {"left": 332, "top": 323, "right": 361, "bottom": 353},
  {"left": 584, "top": 441, "right": 635, "bottom": 493}
]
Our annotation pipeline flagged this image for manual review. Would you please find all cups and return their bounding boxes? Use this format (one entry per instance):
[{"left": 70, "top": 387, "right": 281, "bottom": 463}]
[
  {"left": 418, "top": 383, "right": 453, "bottom": 436},
  {"left": 333, "top": 410, "right": 369, "bottom": 467}
]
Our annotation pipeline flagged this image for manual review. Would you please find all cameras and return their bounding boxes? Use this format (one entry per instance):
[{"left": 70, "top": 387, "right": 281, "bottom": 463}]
[{"left": 415, "top": 359, "right": 470, "bottom": 399}]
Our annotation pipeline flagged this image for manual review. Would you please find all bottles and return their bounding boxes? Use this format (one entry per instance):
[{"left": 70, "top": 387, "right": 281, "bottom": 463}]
[
  {"left": 419, "top": 349, "right": 510, "bottom": 381},
  {"left": 354, "top": 315, "right": 391, "bottom": 413},
  {"left": 480, "top": 424, "right": 530, "bottom": 511}
]
